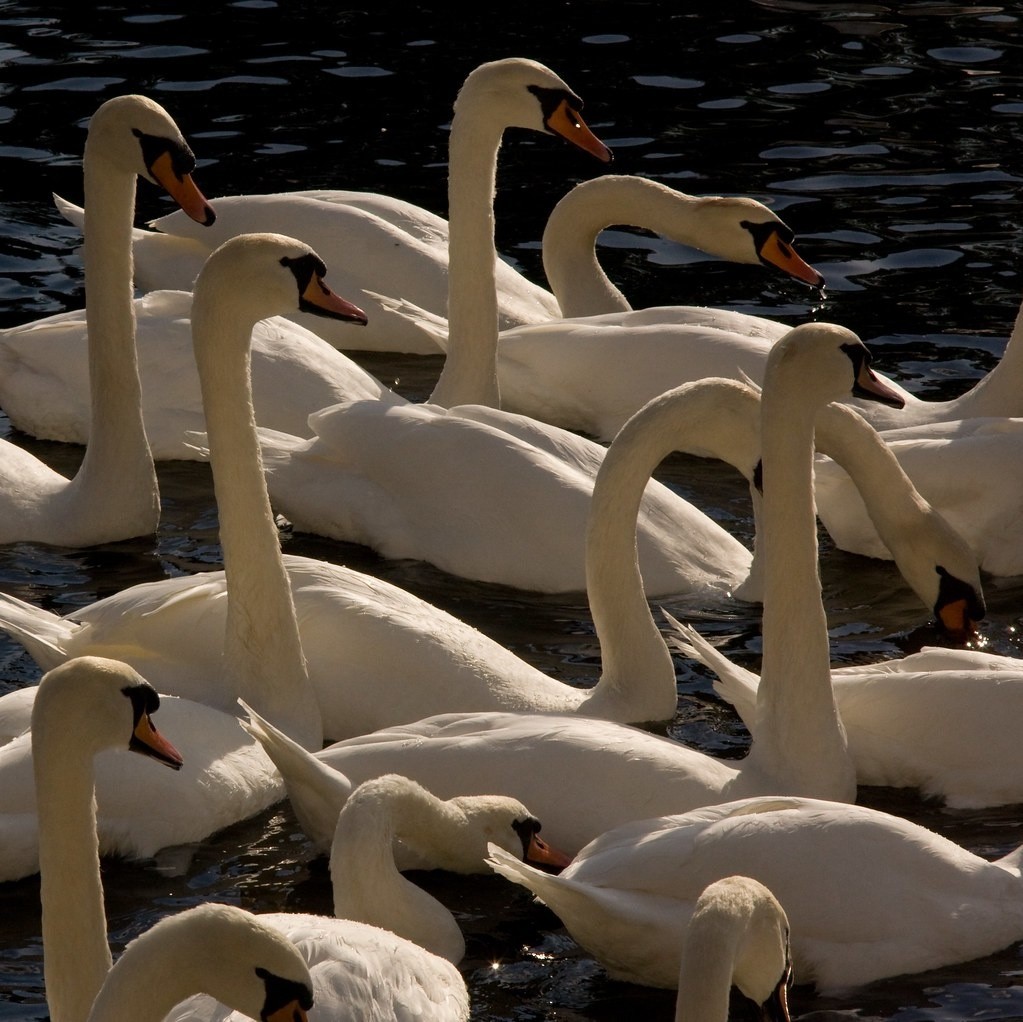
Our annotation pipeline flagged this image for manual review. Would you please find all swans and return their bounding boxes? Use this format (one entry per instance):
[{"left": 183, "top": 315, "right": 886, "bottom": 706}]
[{"left": 0, "top": 52, "right": 1023, "bottom": 1022}]
